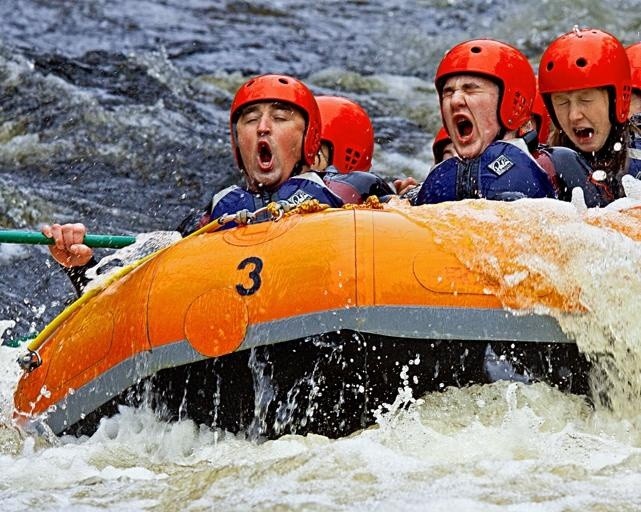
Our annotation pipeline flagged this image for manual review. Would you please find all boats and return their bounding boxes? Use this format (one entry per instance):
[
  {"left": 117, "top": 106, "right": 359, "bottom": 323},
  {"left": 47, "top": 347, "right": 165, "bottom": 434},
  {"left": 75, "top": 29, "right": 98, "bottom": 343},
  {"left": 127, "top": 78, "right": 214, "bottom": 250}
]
[{"left": 11, "top": 203, "right": 641, "bottom": 452}]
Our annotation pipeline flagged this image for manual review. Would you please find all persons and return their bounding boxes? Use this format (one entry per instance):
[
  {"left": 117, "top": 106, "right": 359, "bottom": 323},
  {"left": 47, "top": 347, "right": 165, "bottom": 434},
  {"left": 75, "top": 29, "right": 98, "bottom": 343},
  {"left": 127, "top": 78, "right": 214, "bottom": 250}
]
[
  {"left": 43, "top": 74, "right": 406, "bottom": 298},
  {"left": 393, "top": 29, "right": 640, "bottom": 209}
]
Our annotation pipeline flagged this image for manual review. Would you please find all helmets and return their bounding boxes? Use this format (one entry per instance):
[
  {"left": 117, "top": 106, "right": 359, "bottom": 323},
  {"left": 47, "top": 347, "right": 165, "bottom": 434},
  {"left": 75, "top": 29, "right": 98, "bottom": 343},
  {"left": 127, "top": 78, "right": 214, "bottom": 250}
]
[
  {"left": 230, "top": 74, "right": 322, "bottom": 170},
  {"left": 431, "top": 25, "right": 640, "bottom": 164},
  {"left": 314, "top": 93, "right": 374, "bottom": 172}
]
